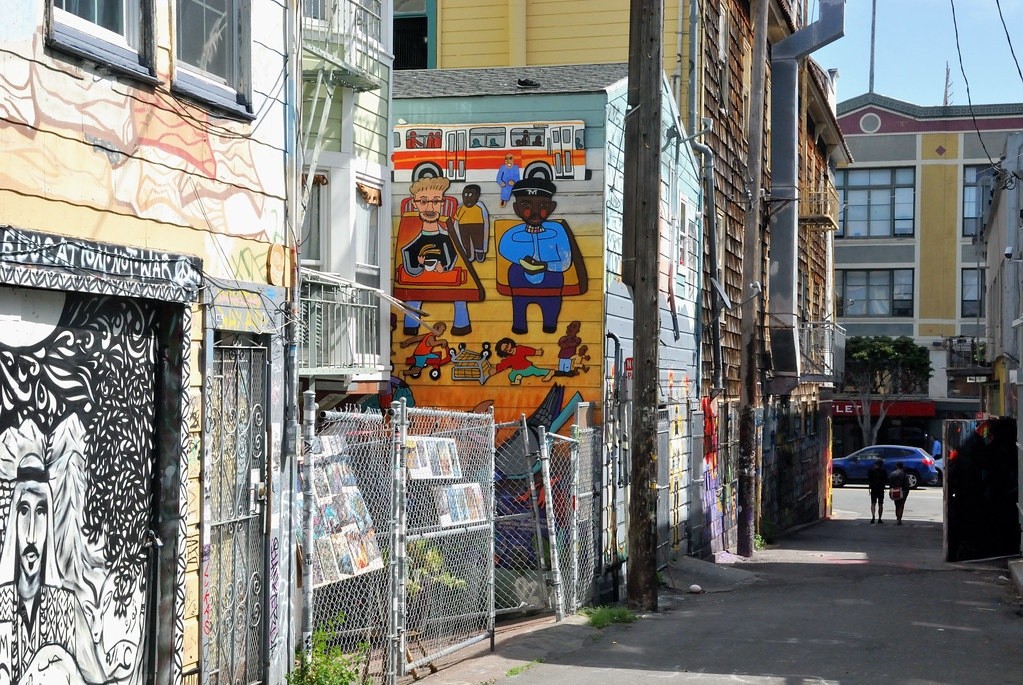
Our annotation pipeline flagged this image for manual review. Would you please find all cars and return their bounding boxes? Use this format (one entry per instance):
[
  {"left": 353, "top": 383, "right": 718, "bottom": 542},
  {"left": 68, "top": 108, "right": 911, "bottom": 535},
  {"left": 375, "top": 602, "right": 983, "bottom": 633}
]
[
  {"left": 832, "top": 444, "right": 939, "bottom": 489},
  {"left": 926, "top": 457, "right": 943, "bottom": 488}
]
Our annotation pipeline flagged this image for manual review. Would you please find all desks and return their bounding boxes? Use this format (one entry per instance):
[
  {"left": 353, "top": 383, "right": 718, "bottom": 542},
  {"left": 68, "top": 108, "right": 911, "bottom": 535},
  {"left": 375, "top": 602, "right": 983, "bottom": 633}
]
[{"left": 361, "top": 630, "right": 436, "bottom": 685}]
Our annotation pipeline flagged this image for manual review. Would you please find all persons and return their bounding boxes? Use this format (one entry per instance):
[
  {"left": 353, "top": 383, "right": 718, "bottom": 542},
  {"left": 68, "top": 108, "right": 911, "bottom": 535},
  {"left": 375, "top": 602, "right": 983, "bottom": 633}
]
[
  {"left": 930, "top": 435, "right": 941, "bottom": 460},
  {"left": 888, "top": 461, "right": 909, "bottom": 525},
  {"left": 867, "top": 459, "right": 887, "bottom": 524}
]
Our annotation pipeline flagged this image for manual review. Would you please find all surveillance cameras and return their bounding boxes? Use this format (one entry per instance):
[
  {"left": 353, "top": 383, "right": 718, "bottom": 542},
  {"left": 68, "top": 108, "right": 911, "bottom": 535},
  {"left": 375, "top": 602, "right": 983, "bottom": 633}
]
[{"left": 1005, "top": 247, "right": 1013, "bottom": 259}]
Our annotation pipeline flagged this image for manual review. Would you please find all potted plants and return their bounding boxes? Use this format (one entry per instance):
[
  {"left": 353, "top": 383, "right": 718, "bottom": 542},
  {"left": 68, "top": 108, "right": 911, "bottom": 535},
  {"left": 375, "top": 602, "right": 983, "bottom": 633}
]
[{"left": 375, "top": 540, "right": 465, "bottom": 632}]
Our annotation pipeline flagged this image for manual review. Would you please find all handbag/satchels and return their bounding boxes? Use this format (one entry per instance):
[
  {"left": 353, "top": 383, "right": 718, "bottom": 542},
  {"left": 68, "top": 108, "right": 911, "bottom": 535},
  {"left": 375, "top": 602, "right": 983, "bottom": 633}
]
[{"left": 889, "top": 486, "right": 903, "bottom": 500}]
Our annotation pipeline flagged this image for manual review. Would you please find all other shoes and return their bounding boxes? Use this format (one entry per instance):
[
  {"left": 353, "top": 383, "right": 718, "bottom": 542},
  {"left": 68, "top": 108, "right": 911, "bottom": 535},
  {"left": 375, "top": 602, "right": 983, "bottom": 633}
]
[
  {"left": 878, "top": 519, "right": 884, "bottom": 523},
  {"left": 897, "top": 520, "right": 902, "bottom": 525},
  {"left": 870, "top": 518, "right": 875, "bottom": 524}
]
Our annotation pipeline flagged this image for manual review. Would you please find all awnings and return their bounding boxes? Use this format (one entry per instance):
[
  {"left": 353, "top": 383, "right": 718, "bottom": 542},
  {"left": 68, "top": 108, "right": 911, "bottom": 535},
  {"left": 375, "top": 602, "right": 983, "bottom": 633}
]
[{"left": 832, "top": 400, "right": 937, "bottom": 417}]
